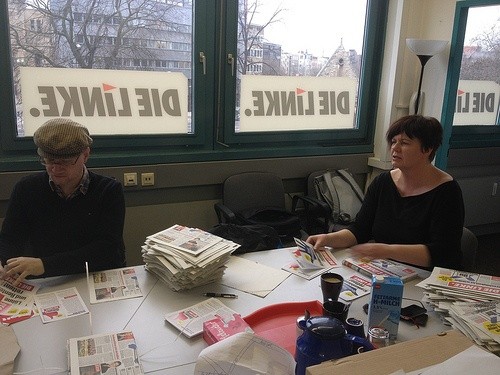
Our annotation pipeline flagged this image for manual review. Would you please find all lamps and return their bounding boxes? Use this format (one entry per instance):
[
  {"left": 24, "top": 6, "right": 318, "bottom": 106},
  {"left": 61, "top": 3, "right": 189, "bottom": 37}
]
[{"left": 406, "top": 38, "right": 449, "bottom": 115}]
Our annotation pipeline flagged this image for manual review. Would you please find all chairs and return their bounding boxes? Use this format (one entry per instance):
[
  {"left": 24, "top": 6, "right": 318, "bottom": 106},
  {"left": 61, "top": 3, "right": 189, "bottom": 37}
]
[
  {"left": 213, "top": 172, "right": 330, "bottom": 251},
  {"left": 305, "top": 170, "right": 366, "bottom": 232}
]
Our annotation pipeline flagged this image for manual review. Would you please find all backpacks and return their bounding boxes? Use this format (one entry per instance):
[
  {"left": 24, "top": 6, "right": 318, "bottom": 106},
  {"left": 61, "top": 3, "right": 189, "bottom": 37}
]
[{"left": 313, "top": 168, "right": 365, "bottom": 233}]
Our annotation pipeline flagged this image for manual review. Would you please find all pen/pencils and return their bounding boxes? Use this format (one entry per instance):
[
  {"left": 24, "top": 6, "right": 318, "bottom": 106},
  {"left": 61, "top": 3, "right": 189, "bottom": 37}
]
[{"left": 200, "top": 293, "right": 237, "bottom": 298}]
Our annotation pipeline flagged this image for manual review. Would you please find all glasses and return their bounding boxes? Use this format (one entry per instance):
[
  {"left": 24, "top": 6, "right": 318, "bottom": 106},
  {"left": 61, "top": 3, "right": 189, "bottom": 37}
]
[{"left": 40, "top": 155, "right": 81, "bottom": 168}]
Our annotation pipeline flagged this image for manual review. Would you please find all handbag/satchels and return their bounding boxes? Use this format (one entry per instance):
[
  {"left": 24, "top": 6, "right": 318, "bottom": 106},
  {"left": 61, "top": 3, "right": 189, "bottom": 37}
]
[
  {"left": 207, "top": 224, "right": 282, "bottom": 257},
  {"left": 235, "top": 205, "right": 302, "bottom": 244}
]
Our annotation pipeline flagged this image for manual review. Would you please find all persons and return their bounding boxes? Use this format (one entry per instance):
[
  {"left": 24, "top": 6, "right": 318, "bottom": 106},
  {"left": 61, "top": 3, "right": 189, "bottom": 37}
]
[
  {"left": 101, "top": 361, "right": 121, "bottom": 372},
  {"left": 0, "top": 118, "right": 125, "bottom": 287},
  {"left": 305, "top": 115, "right": 465, "bottom": 273},
  {"left": 189, "top": 238, "right": 200, "bottom": 243}
]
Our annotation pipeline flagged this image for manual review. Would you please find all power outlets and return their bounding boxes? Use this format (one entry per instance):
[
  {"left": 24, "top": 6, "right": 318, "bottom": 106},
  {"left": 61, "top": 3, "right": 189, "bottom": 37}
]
[{"left": 140, "top": 172, "right": 155, "bottom": 185}]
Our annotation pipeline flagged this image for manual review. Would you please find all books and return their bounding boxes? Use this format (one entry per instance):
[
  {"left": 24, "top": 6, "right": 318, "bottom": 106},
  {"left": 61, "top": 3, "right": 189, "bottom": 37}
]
[
  {"left": 164, "top": 298, "right": 240, "bottom": 339},
  {"left": 342, "top": 255, "right": 421, "bottom": 283}
]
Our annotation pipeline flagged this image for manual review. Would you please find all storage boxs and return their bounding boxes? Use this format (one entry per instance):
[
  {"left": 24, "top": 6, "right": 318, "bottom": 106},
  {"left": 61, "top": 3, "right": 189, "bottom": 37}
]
[{"left": 306, "top": 329, "right": 500, "bottom": 375}]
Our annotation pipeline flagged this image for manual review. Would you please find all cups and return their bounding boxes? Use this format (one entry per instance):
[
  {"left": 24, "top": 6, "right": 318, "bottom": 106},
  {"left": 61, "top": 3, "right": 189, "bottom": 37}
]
[
  {"left": 320, "top": 272, "right": 344, "bottom": 302},
  {"left": 344, "top": 317, "right": 365, "bottom": 350},
  {"left": 322, "top": 301, "right": 348, "bottom": 323},
  {"left": 367, "top": 326, "right": 390, "bottom": 350}
]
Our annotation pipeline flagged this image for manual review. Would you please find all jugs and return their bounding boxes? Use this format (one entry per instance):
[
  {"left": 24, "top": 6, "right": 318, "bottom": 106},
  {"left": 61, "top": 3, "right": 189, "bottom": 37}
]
[{"left": 295, "top": 316, "right": 375, "bottom": 375}]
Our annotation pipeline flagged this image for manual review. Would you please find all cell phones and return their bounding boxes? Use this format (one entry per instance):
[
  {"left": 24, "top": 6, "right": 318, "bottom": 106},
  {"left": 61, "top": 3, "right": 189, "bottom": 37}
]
[{"left": 400, "top": 304, "right": 427, "bottom": 320}]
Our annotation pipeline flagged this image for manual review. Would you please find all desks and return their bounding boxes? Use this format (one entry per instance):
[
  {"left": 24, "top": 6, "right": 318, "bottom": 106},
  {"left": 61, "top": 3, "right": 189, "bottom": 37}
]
[{"left": 0, "top": 247, "right": 500, "bottom": 375}]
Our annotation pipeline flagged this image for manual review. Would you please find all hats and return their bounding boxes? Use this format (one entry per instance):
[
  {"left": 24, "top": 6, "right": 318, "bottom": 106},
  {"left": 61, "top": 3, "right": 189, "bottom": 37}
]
[{"left": 33, "top": 118, "right": 94, "bottom": 160}]
